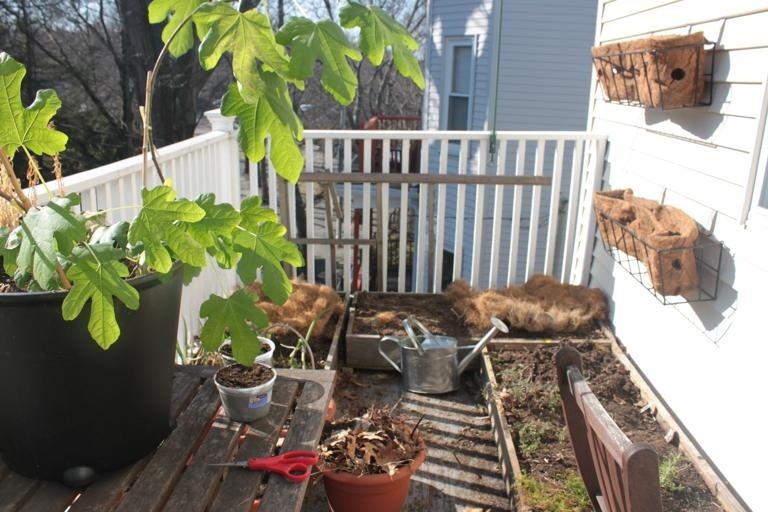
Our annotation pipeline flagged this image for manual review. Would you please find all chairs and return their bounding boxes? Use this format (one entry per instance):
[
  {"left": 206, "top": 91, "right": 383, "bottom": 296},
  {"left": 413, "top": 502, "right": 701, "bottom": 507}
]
[{"left": 550, "top": 344, "right": 662, "bottom": 512}]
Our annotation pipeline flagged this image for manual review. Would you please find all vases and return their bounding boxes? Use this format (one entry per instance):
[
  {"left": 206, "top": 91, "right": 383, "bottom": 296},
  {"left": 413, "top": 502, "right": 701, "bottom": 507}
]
[
  {"left": 217, "top": 337, "right": 275, "bottom": 367},
  {"left": 214, "top": 362, "right": 276, "bottom": 422}
]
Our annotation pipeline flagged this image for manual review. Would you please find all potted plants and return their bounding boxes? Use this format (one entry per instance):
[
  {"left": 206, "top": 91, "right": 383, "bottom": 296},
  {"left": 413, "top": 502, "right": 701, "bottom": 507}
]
[
  {"left": 1, "top": 0, "right": 431, "bottom": 488},
  {"left": 316, "top": 418, "right": 428, "bottom": 511}
]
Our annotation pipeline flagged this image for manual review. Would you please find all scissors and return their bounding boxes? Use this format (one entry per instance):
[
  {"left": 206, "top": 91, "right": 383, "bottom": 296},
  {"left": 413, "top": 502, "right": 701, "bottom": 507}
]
[{"left": 207, "top": 451, "right": 319, "bottom": 481}]
